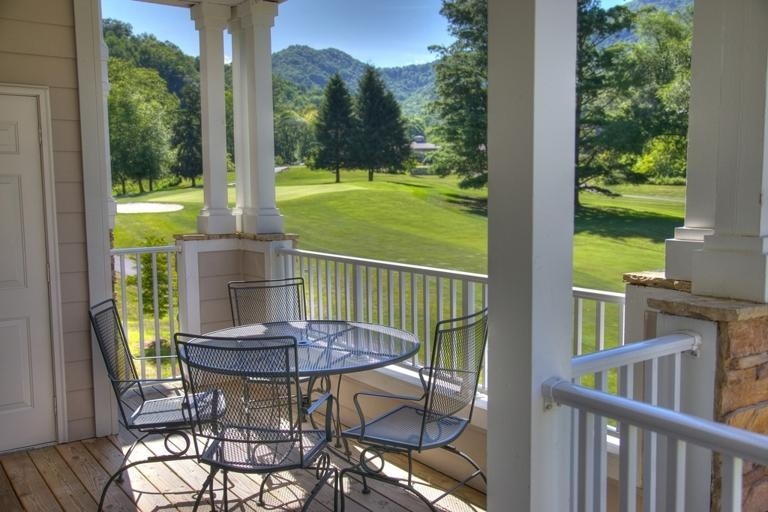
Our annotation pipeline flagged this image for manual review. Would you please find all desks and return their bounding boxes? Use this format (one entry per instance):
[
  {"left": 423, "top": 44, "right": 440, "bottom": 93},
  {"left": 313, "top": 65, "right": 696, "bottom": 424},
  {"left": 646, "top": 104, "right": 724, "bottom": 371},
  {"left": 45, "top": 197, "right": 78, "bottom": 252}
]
[{"left": 182, "top": 319, "right": 420, "bottom": 506}]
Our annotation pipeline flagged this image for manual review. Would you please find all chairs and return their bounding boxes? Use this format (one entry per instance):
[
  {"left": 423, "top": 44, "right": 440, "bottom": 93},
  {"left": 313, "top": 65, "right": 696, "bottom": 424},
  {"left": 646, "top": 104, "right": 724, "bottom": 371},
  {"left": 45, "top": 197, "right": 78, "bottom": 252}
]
[
  {"left": 88, "top": 298, "right": 228, "bottom": 512},
  {"left": 227, "top": 281, "right": 340, "bottom": 453},
  {"left": 170, "top": 329, "right": 341, "bottom": 512},
  {"left": 342, "top": 305, "right": 488, "bottom": 511}
]
